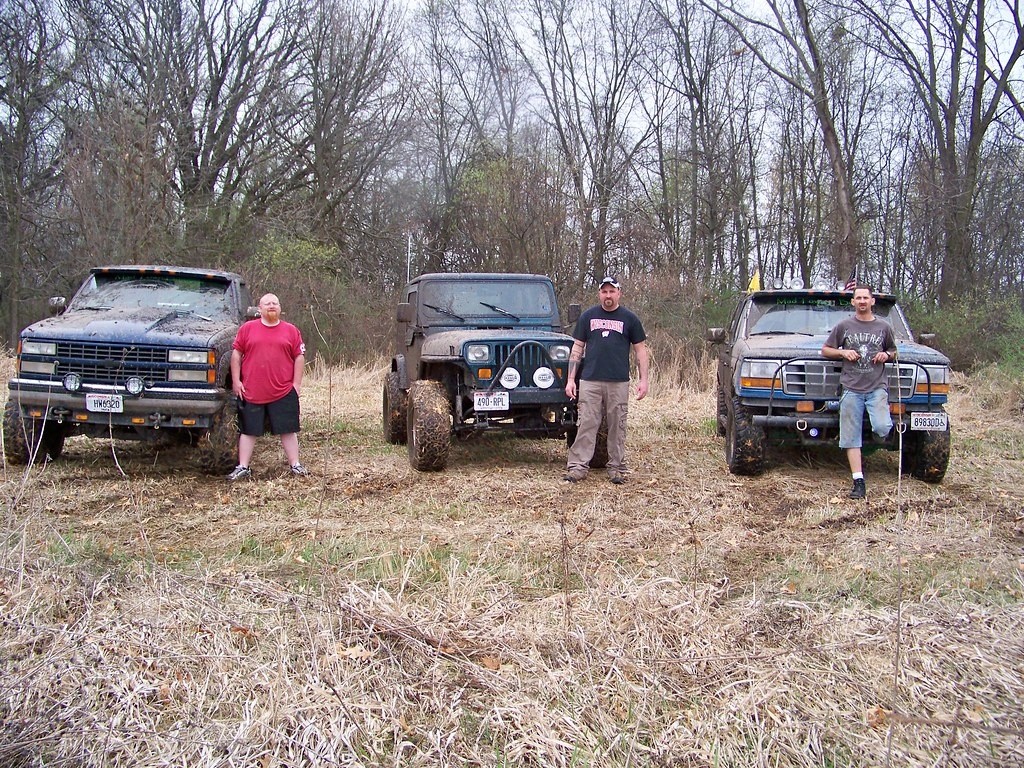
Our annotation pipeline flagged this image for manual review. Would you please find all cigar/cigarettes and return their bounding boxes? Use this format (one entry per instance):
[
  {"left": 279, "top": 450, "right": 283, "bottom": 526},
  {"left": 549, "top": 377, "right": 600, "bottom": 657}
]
[{"left": 570, "top": 397, "right": 573, "bottom": 401}]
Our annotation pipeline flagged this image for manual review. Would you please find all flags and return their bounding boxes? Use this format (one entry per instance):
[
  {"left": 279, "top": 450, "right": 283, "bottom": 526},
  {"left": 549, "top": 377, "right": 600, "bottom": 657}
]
[
  {"left": 746, "top": 271, "right": 759, "bottom": 296},
  {"left": 844, "top": 264, "right": 855, "bottom": 291}
]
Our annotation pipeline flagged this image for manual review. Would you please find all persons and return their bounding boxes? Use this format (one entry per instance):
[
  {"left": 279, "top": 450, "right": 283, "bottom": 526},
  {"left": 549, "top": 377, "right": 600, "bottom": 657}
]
[
  {"left": 563, "top": 276, "right": 649, "bottom": 484},
  {"left": 224, "top": 292, "right": 305, "bottom": 481},
  {"left": 821, "top": 284, "right": 898, "bottom": 499}
]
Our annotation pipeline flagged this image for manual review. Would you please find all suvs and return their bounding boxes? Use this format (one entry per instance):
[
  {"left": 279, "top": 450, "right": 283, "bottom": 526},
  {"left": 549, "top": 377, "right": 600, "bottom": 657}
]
[
  {"left": 3, "top": 258, "right": 261, "bottom": 480},
  {"left": 697, "top": 272, "right": 950, "bottom": 485},
  {"left": 371, "top": 231, "right": 609, "bottom": 477}
]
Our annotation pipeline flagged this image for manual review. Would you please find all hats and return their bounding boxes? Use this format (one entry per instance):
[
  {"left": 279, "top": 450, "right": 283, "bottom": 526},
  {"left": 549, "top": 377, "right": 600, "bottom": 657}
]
[{"left": 598, "top": 276, "right": 621, "bottom": 291}]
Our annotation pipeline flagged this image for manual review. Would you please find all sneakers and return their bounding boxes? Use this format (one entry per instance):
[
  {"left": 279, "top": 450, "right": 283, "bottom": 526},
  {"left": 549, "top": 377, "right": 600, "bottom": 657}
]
[
  {"left": 849, "top": 479, "right": 865, "bottom": 499},
  {"left": 606, "top": 465, "right": 628, "bottom": 484},
  {"left": 563, "top": 469, "right": 588, "bottom": 482},
  {"left": 224, "top": 465, "right": 253, "bottom": 481},
  {"left": 872, "top": 432, "right": 886, "bottom": 443},
  {"left": 290, "top": 464, "right": 308, "bottom": 476}
]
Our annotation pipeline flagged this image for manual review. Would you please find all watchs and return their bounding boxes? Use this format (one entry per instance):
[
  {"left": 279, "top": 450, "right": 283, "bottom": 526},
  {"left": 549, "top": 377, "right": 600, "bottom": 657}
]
[{"left": 885, "top": 351, "right": 891, "bottom": 358}]
